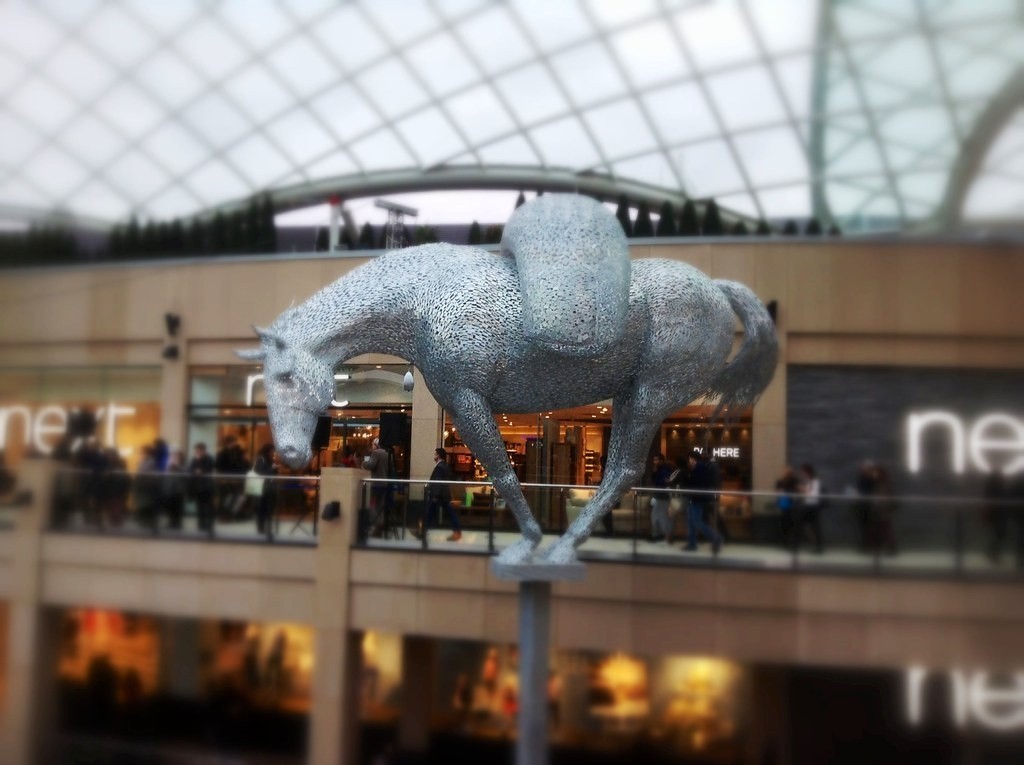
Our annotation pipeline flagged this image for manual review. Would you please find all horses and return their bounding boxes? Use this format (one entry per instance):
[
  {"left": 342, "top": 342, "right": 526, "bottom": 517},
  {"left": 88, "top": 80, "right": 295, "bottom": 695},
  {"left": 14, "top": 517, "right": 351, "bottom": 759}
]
[{"left": 231, "top": 191, "right": 780, "bottom": 565}]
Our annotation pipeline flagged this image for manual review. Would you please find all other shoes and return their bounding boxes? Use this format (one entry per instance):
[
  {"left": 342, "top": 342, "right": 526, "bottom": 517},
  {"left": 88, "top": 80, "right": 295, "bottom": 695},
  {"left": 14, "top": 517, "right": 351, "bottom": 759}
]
[
  {"left": 710, "top": 533, "right": 724, "bottom": 555},
  {"left": 447, "top": 531, "right": 461, "bottom": 541},
  {"left": 409, "top": 528, "right": 422, "bottom": 539},
  {"left": 683, "top": 541, "right": 698, "bottom": 552}
]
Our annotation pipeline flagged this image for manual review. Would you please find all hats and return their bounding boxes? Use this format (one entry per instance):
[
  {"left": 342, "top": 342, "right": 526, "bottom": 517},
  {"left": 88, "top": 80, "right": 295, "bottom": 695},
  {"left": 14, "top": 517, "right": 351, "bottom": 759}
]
[{"left": 373, "top": 437, "right": 382, "bottom": 449}]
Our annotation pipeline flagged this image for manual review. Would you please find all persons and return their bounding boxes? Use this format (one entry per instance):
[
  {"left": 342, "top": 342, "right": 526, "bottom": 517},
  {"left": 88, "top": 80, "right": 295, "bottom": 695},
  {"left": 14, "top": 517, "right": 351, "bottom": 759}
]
[
  {"left": 43, "top": 402, "right": 464, "bottom": 546},
  {"left": 642, "top": 447, "right": 1024, "bottom": 573}
]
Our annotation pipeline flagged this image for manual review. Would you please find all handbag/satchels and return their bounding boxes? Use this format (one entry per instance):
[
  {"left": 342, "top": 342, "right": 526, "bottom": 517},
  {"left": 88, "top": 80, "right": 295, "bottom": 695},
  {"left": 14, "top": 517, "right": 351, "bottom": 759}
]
[
  {"left": 388, "top": 466, "right": 398, "bottom": 489},
  {"left": 243, "top": 456, "right": 267, "bottom": 496}
]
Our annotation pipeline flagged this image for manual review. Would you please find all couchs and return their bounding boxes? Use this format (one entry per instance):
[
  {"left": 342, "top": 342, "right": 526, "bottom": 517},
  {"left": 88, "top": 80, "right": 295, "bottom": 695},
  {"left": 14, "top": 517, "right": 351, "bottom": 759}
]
[
  {"left": 668, "top": 490, "right": 751, "bottom": 541},
  {"left": 565, "top": 488, "right": 649, "bottom": 535},
  {"left": 446, "top": 483, "right": 518, "bottom": 529}
]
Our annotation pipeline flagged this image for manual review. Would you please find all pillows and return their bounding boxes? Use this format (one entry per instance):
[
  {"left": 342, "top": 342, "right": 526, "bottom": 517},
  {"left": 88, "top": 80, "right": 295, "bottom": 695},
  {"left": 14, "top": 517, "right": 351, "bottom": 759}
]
[
  {"left": 465, "top": 486, "right": 483, "bottom": 505},
  {"left": 485, "top": 486, "right": 499, "bottom": 496},
  {"left": 569, "top": 489, "right": 590, "bottom": 506}
]
